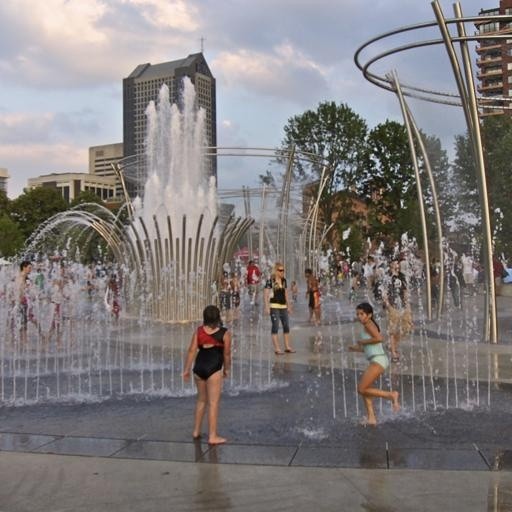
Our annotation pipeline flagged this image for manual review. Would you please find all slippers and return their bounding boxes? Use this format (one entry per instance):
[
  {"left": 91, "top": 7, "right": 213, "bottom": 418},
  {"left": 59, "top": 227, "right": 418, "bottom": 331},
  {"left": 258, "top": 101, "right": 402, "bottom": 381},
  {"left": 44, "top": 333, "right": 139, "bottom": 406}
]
[
  {"left": 275, "top": 350, "right": 285, "bottom": 355},
  {"left": 285, "top": 349, "right": 297, "bottom": 353},
  {"left": 391, "top": 357, "right": 399, "bottom": 363}
]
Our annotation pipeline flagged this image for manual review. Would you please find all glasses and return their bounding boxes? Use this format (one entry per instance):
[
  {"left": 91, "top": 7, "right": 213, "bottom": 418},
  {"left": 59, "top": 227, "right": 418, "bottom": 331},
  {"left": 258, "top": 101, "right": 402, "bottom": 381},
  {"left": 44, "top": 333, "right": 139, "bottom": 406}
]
[{"left": 276, "top": 269, "right": 284, "bottom": 272}]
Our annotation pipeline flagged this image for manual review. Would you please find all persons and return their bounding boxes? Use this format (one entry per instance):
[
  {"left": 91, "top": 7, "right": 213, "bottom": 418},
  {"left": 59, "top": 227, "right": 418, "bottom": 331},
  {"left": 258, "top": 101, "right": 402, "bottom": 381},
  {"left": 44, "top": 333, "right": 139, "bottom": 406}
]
[
  {"left": 5, "top": 261, "right": 121, "bottom": 351},
  {"left": 291, "top": 280, "right": 297, "bottom": 300},
  {"left": 493, "top": 253, "right": 503, "bottom": 295},
  {"left": 428, "top": 256, "right": 484, "bottom": 317},
  {"left": 400, "top": 253, "right": 414, "bottom": 281},
  {"left": 263, "top": 263, "right": 296, "bottom": 355},
  {"left": 346, "top": 303, "right": 398, "bottom": 425},
  {"left": 330, "top": 252, "right": 386, "bottom": 301},
  {"left": 304, "top": 268, "right": 321, "bottom": 324},
  {"left": 218, "top": 271, "right": 241, "bottom": 310},
  {"left": 245, "top": 260, "right": 261, "bottom": 305},
  {"left": 501, "top": 259, "right": 511, "bottom": 285},
  {"left": 382, "top": 258, "right": 413, "bottom": 363},
  {"left": 289, "top": 281, "right": 298, "bottom": 304},
  {"left": 182, "top": 305, "right": 232, "bottom": 444}
]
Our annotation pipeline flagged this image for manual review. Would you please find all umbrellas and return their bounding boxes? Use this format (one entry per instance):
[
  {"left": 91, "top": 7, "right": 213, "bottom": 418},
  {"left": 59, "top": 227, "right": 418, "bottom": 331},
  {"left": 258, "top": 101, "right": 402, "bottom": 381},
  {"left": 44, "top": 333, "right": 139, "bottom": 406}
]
[{"left": 233, "top": 245, "right": 259, "bottom": 261}]
